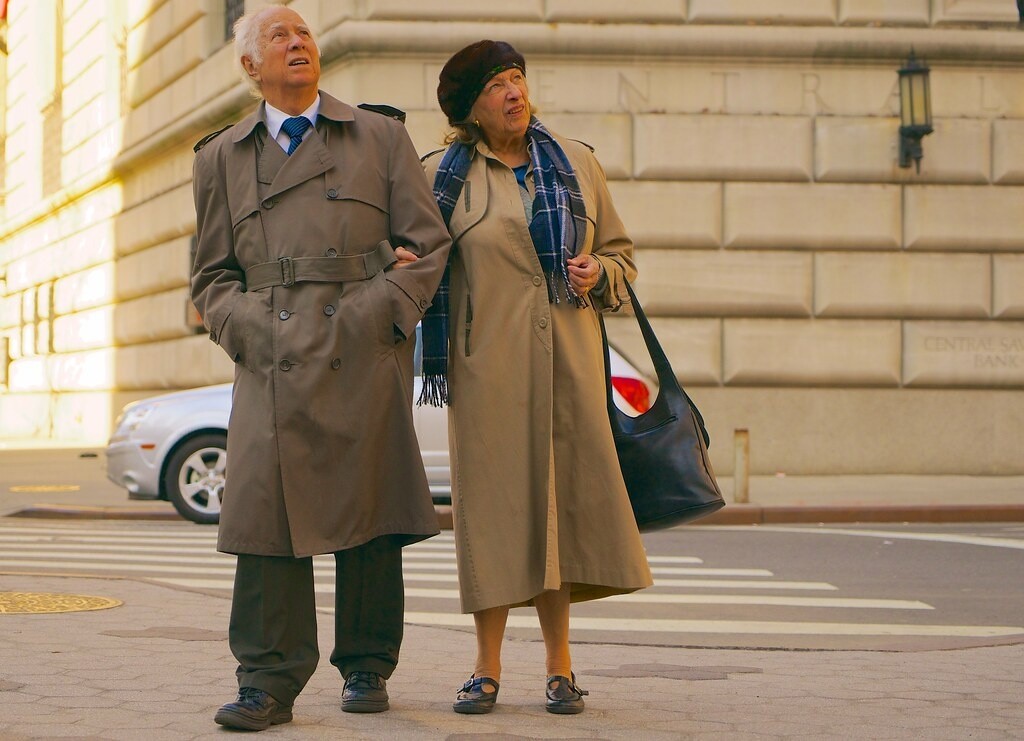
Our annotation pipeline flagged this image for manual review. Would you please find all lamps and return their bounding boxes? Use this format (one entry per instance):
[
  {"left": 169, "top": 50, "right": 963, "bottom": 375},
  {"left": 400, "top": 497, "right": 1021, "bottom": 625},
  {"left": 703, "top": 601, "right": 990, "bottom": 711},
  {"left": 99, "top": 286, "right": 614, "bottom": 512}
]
[{"left": 896, "top": 43, "right": 932, "bottom": 175}]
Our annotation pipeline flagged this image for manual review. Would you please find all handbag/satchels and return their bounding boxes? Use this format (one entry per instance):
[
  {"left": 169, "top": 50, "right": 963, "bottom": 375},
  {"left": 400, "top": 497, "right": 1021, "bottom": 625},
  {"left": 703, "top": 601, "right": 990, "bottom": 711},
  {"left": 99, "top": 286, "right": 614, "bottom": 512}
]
[{"left": 598, "top": 274, "right": 726, "bottom": 533}]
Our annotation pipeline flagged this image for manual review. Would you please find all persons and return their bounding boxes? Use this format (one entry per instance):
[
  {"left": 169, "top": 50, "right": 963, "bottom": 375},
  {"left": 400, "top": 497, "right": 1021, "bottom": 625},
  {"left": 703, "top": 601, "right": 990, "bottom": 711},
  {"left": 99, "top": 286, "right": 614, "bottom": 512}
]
[
  {"left": 189, "top": 6, "right": 453, "bottom": 730},
  {"left": 394, "top": 39, "right": 638, "bottom": 713}
]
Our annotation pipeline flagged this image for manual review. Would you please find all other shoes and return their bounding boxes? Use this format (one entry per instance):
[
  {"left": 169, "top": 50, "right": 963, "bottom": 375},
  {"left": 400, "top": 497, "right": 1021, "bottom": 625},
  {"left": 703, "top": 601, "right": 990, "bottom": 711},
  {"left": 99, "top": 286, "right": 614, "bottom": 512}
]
[
  {"left": 452, "top": 673, "right": 499, "bottom": 713},
  {"left": 545, "top": 671, "right": 590, "bottom": 714}
]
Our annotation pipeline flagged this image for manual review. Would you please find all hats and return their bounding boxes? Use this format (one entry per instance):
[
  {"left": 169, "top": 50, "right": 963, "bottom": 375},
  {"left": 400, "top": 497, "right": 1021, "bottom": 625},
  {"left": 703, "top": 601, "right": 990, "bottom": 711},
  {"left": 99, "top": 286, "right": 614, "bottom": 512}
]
[{"left": 437, "top": 39, "right": 526, "bottom": 123}]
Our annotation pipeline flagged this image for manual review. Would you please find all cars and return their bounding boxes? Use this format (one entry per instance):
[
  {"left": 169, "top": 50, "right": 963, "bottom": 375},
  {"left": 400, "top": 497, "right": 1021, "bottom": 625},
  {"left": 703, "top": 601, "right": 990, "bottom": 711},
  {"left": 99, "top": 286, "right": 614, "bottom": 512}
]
[{"left": 104, "top": 316, "right": 667, "bottom": 527}]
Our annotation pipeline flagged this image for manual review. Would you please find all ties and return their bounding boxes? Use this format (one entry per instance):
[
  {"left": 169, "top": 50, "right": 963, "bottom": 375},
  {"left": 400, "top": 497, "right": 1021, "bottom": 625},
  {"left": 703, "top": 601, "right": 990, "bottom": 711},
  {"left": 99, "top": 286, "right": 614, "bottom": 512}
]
[{"left": 281, "top": 116, "right": 311, "bottom": 156}]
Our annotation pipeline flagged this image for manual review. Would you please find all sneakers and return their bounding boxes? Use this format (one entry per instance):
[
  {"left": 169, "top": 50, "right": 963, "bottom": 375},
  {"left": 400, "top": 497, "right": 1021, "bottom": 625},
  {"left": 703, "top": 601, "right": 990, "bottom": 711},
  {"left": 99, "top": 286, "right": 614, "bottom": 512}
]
[
  {"left": 340, "top": 671, "right": 390, "bottom": 713},
  {"left": 214, "top": 687, "right": 293, "bottom": 728}
]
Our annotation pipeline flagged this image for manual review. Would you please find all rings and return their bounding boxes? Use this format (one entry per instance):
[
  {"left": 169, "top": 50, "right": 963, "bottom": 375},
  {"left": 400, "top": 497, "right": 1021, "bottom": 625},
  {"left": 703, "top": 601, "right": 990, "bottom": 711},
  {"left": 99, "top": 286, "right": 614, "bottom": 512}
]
[{"left": 583, "top": 288, "right": 588, "bottom": 295}]
